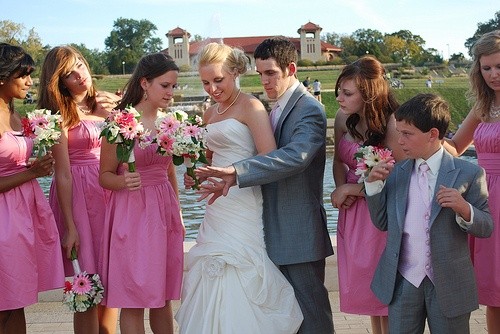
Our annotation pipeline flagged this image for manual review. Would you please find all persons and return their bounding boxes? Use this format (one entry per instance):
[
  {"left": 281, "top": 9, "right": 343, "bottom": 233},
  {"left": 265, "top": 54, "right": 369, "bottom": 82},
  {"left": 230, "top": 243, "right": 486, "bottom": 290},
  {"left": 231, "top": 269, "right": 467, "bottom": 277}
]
[
  {"left": 312, "top": 79, "right": 322, "bottom": 103},
  {"left": 99, "top": 54, "right": 187, "bottom": 334},
  {"left": 35, "top": 44, "right": 121, "bottom": 334},
  {"left": 0, "top": 40, "right": 57, "bottom": 334},
  {"left": 330, "top": 30, "right": 500, "bottom": 334},
  {"left": 426, "top": 75, "right": 433, "bottom": 88},
  {"left": 115, "top": 88, "right": 122, "bottom": 96},
  {"left": 303, "top": 76, "right": 312, "bottom": 93},
  {"left": 24, "top": 92, "right": 33, "bottom": 104},
  {"left": 183, "top": 34, "right": 336, "bottom": 334}
]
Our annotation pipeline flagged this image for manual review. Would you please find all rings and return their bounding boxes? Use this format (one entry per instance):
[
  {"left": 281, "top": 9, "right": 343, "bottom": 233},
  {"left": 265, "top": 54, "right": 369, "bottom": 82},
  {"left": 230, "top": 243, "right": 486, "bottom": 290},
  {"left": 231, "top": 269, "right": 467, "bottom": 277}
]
[{"left": 211, "top": 182, "right": 216, "bottom": 187}]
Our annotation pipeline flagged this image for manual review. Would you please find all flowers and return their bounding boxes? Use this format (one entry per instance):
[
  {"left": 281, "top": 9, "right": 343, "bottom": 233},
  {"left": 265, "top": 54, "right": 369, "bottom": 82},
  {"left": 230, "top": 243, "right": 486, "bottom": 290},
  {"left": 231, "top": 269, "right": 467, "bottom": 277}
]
[
  {"left": 351, "top": 143, "right": 395, "bottom": 194},
  {"left": 102, "top": 107, "right": 151, "bottom": 172},
  {"left": 149, "top": 110, "right": 211, "bottom": 192},
  {"left": 62, "top": 246, "right": 104, "bottom": 313},
  {"left": 22, "top": 108, "right": 64, "bottom": 159}
]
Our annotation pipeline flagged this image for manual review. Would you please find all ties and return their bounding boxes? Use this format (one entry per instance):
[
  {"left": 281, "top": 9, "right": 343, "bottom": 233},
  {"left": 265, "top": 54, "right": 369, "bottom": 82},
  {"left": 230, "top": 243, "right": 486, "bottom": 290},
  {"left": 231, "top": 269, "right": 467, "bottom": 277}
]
[
  {"left": 417, "top": 162, "right": 430, "bottom": 208},
  {"left": 269, "top": 101, "right": 281, "bottom": 134}
]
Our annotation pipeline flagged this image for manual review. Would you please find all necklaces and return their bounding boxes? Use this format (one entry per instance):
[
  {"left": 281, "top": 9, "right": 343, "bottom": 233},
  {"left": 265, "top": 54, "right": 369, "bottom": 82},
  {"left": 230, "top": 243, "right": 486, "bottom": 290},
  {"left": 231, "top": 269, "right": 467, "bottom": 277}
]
[
  {"left": 216, "top": 89, "right": 241, "bottom": 114},
  {"left": 489, "top": 97, "right": 500, "bottom": 118},
  {"left": 73, "top": 100, "right": 92, "bottom": 113}
]
